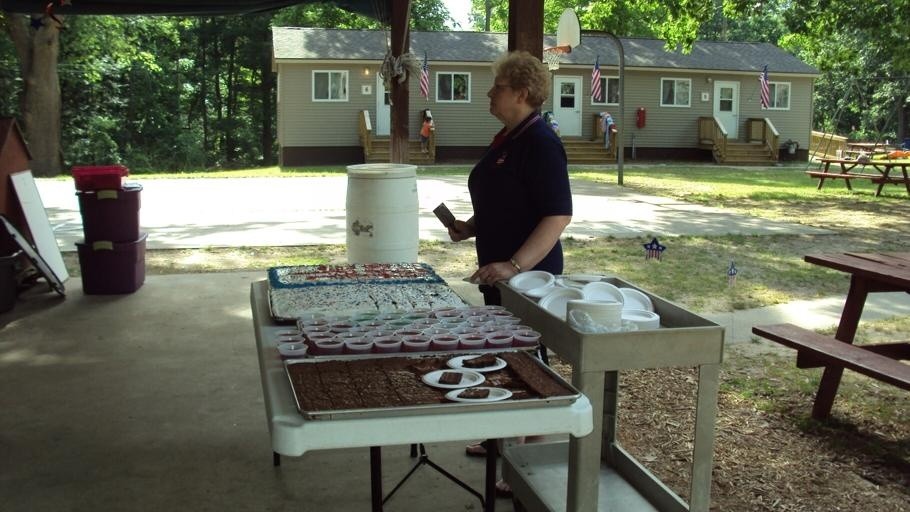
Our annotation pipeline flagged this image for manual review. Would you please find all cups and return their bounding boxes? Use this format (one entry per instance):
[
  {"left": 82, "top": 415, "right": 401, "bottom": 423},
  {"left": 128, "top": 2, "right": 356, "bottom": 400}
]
[{"left": 273, "top": 306, "right": 543, "bottom": 362}]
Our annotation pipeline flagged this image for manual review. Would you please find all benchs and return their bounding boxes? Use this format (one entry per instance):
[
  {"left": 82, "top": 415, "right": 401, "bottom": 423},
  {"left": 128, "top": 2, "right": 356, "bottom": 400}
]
[
  {"left": 751, "top": 323, "right": 910, "bottom": 419},
  {"left": 847, "top": 172, "right": 910, "bottom": 179},
  {"left": 804, "top": 170, "right": 883, "bottom": 180}
]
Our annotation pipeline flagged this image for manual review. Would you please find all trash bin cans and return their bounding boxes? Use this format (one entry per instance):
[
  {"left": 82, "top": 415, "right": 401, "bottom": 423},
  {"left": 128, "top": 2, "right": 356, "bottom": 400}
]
[{"left": 784, "top": 141, "right": 799, "bottom": 161}]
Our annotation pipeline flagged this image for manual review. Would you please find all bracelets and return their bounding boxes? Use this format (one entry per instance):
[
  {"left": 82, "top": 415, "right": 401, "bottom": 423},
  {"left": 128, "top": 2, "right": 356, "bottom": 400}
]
[{"left": 509, "top": 258, "right": 521, "bottom": 272}]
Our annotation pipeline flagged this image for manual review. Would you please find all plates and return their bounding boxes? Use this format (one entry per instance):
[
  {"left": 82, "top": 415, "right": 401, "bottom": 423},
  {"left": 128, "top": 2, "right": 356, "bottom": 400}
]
[
  {"left": 446, "top": 355, "right": 508, "bottom": 373},
  {"left": 423, "top": 369, "right": 486, "bottom": 389},
  {"left": 508, "top": 271, "right": 660, "bottom": 333},
  {"left": 444, "top": 386, "right": 513, "bottom": 402}
]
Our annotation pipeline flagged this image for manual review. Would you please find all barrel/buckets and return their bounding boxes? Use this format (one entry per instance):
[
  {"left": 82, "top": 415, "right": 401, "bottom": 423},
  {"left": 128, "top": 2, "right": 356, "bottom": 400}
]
[{"left": 346, "top": 162, "right": 420, "bottom": 265}]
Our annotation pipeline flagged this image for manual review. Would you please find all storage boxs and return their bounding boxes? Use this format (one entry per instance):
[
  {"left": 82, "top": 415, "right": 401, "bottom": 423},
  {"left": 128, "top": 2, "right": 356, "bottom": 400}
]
[
  {"left": 75, "top": 231, "right": 149, "bottom": 295},
  {"left": 75, "top": 184, "right": 143, "bottom": 243},
  {"left": 72, "top": 166, "right": 128, "bottom": 191}
]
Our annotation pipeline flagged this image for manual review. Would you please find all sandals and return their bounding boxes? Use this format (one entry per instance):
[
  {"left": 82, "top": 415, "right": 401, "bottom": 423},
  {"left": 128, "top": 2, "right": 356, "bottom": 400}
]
[
  {"left": 466, "top": 439, "right": 514, "bottom": 457},
  {"left": 494, "top": 474, "right": 518, "bottom": 498}
]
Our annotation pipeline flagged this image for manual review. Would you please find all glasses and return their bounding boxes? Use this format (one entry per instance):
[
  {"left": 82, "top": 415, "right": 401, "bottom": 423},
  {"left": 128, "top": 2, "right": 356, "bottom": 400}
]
[{"left": 494, "top": 84, "right": 520, "bottom": 90}]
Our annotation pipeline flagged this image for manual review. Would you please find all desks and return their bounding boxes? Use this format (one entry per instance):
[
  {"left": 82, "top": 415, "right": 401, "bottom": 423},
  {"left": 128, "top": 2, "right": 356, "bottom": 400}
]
[
  {"left": 803, "top": 251, "right": 910, "bottom": 419},
  {"left": 816, "top": 159, "right": 910, "bottom": 197},
  {"left": 250, "top": 278, "right": 595, "bottom": 512}
]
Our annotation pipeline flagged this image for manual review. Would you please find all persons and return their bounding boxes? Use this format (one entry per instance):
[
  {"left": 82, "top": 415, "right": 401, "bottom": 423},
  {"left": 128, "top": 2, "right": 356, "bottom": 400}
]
[
  {"left": 449, "top": 51, "right": 573, "bottom": 500},
  {"left": 419, "top": 109, "right": 435, "bottom": 153}
]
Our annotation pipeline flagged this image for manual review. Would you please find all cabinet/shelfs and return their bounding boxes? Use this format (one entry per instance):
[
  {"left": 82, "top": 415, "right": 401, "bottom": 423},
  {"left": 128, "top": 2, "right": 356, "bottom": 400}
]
[{"left": 498, "top": 274, "right": 726, "bottom": 512}]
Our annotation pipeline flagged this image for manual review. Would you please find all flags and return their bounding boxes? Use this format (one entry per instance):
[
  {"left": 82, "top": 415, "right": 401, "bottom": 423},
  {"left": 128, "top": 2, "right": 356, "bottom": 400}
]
[
  {"left": 420, "top": 52, "right": 429, "bottom": 101},
  {"left": 760, "top": 65, "right": 769, "bottom": 108},
  {"left": 591, "top": 59, "right": 602, "bottom": 101}
]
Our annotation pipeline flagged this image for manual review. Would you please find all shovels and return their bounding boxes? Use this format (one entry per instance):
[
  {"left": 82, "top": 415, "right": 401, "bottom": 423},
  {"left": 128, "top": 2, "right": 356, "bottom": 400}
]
[{"left": 433, "top": 202, "right": 460, "bottom": 233}]
